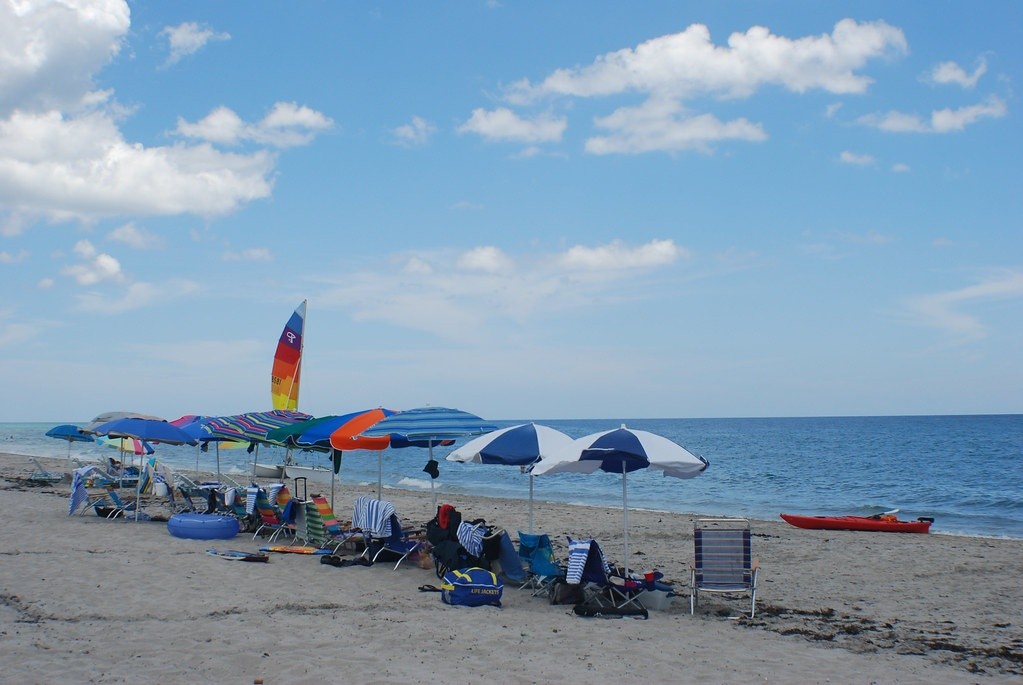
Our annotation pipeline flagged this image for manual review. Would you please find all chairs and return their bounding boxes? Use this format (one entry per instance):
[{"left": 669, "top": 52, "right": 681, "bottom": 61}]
[
  {"left": 27, "top": 458, "right": 64, "bottom": 488},
  {"left": 690, "top": 519, "right": 759, "bottom": 619},
  {"left": 71, "top": 457, "right": 676, "bottom": 616}
]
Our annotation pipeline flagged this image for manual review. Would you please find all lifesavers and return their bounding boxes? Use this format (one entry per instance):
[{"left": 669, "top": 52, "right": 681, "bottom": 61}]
[{"left": 163, "top": 512, "right": 238, "bottom": 539}]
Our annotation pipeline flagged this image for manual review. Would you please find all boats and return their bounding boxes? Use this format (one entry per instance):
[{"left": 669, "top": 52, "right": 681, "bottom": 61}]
[
  {"left": 279, "top": 464, "right": 334, "bottom": 481},
  {"left": 249, "top": 462, "right": 282, "bottom": 478},
  {"left": 779, "top": 510, "right": 936, "bottom": 534}
]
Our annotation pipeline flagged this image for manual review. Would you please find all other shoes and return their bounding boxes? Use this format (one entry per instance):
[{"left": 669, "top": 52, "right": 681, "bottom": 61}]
[
  {"left": 319, "top": 555, "right": 341, "bottom": 566},
  {"left": 353, "top": 556, "right": 374, "bottom": 566}
]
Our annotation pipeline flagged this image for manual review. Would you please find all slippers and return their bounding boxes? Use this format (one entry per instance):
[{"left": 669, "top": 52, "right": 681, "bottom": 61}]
[{"left": 418, "top": 584, "right": 442, "bottom": 592}]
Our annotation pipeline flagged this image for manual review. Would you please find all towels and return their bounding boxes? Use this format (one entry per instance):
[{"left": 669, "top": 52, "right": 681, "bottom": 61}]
[
  {"left": 68, "top": 471, "right": 88, "bottom": 517},
  {"left": 455, "top": 521, "right": 487, "bottom": 558},
  {"left": 566, "top": 538, "right": 611, "bottom": 585},
  {"left": 267, "top": 483, "right": 287, "bottom": 507},
  {"left": 245, "top": 486, "right": 259, "bottom": 514},
  {"left": 350, "top": 495, "right": 394, "bottom": 537}
]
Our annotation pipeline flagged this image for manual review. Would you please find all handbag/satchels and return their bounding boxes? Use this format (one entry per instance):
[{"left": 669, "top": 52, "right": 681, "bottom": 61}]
[
  {"left": 566, "top": 582, "right": 606, "bottom": 617},
  {"left": 282, "top": 497, "right": 300, "bottom": 526},
  {"left": 418, "top": 549, "right": 435, "bottom": 570},
  {"left": 439, "top": 565, "right": 506, "bottom": 608},
  {"left": 551, "top": 581, "right": 574, "bottom": 605}
]
